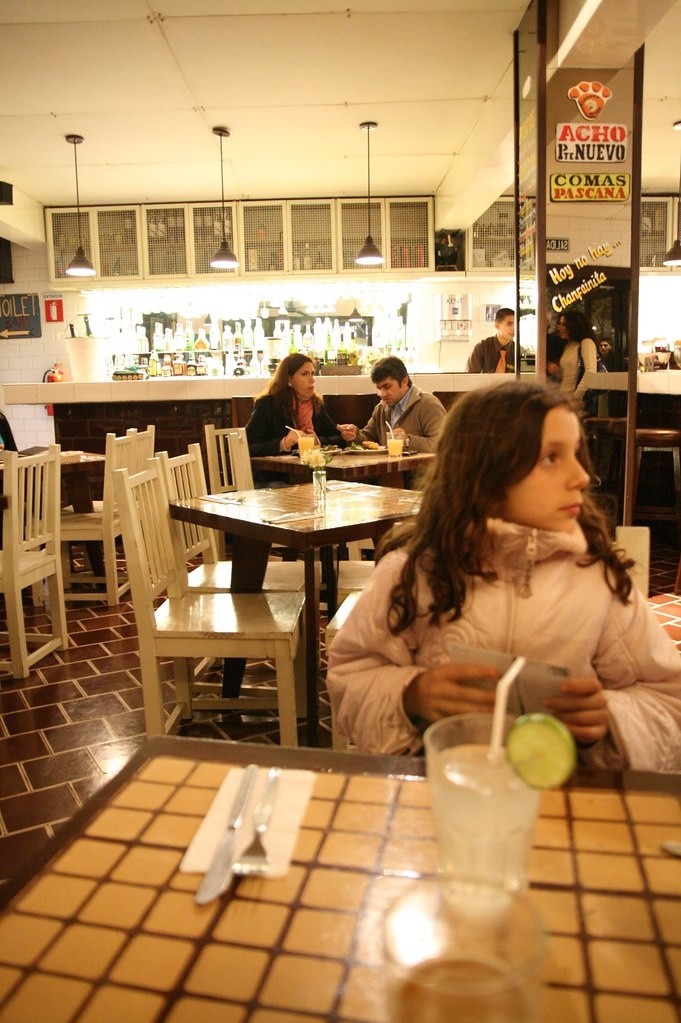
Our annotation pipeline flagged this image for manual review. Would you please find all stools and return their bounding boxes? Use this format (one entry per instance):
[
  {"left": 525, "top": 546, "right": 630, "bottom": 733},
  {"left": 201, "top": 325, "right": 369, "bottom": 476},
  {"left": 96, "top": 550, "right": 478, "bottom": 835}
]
[{"left": 623, "top": 426, "right": 681, "bottom": 555}]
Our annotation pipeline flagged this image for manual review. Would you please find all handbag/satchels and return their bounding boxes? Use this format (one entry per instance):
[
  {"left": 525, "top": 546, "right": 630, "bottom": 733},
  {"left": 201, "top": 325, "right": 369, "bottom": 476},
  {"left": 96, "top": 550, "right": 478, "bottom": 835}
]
[{"left": 576, "top": 346, "right": 610, "bottom": 398}]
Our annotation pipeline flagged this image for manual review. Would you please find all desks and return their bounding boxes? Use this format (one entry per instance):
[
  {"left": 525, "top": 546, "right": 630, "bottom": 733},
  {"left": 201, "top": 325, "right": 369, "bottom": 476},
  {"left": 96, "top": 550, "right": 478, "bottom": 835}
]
[
  {"left": 1, "top": 448, "right": 111, "bottom": 601},
  {"left": 168, "top": 481, "right": 425, "bottom": 746},
  {"left": 0, "top": 736, "right": 681, "bottom": 1023},
  {"left": 248, "top": 442, "right": 434, "bottom": 583}
]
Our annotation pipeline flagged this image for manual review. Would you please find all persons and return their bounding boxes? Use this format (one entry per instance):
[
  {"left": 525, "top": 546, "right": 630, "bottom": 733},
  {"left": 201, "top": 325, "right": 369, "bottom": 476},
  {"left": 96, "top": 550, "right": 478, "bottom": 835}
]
[
  {"left": 467, "top": 306, "right": 523, "bottom": 380},
  {"left": 244, "top": 354, "right": 345, "bottom": 489},
  {"left": 333, "top": 355, "right": 448, "bottom": 490},
  {"left": 326, "top": 379, "right": 681, "bottom": 793},
  {"left": 543, "top": 305, "right": 681, "bottom": 408}
]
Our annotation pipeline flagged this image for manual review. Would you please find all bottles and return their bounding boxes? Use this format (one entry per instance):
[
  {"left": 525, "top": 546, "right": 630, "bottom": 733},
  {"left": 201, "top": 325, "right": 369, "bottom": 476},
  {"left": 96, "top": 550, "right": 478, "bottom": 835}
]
[
  {"left": 94, "top": 207, "right": 326, "bottom": 276},
  {"left": 119, "top": 317, "right": 359, "bottom": 376}
]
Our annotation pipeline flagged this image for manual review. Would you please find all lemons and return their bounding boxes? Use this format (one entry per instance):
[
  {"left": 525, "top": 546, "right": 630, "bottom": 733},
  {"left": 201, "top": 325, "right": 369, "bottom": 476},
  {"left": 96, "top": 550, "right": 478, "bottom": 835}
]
[{"left": 504, "top": 713, "right": 576, "bottom": 789}]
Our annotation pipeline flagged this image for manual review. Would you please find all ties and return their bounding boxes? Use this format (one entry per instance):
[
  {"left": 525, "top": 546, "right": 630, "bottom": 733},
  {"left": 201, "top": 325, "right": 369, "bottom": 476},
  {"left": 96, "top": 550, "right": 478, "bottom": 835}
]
[{"left": 494, "top": 351, "right": 507, "bottom": 373}]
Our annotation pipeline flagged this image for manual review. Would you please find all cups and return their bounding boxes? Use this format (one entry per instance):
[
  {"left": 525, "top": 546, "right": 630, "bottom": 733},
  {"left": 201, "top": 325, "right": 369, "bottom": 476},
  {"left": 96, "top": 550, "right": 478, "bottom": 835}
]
[
  {"left": 385, "top": 432, "right": 403, "bottom": 458},
  {"left": 423, "top": 711, "right": 538, "bottom": 919},
  {"left": 374, "top": 872, "right": 552, "bottom": 1022},
  {"left": 297, "top": 433, "right": 315, "bottom": 457}
]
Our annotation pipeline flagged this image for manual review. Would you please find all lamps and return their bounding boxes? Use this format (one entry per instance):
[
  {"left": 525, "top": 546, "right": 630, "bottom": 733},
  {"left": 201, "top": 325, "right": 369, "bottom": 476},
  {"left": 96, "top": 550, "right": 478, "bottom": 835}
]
[
  {"left": 64, "top": 134, "right": 94, "bottom": 276},
  {"left": 211, "top": 126, "right": 240, "bottom": 267},
  {"left": 349, "top": 298, "right": 364, "bottom": 324},
  {"left": 357, "top": 120, "right": 385, "bottom": 266},
  {"left": 663, "top": 119, "right": 681, "bottom": 267},
  {"left": 204, "top": 311, "right": 211, "bottom": 325}
]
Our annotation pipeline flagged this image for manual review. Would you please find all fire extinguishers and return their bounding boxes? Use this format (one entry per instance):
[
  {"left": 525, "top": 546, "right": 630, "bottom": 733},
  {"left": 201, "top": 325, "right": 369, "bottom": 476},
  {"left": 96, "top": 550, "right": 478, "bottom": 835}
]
[{"left": 42, "top": 363, "right": 64, "bottom": 416}]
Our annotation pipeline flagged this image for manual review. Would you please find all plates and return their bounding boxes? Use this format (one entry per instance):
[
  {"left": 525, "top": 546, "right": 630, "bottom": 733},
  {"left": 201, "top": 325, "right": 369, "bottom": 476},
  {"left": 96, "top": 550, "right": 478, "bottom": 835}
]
[
  {"left": 319, "top": 448, "right": 343, "bottom": 456},
  {"left": 343, "top": 445, "right": 389, "bottom": 454}
]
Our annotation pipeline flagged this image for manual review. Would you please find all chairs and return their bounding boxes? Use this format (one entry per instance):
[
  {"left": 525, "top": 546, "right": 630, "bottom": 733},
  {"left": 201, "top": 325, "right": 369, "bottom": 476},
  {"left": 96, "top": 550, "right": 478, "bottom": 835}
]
[{"left": 0, "top": 421, "right": 380, "bottom": 748}]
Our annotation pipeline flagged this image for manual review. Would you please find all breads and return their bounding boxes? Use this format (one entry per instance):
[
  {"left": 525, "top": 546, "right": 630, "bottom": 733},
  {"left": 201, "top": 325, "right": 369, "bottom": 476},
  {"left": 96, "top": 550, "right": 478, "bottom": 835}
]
[{"left": 361, "top": 440, "right": 380, "bottom": 450}]
